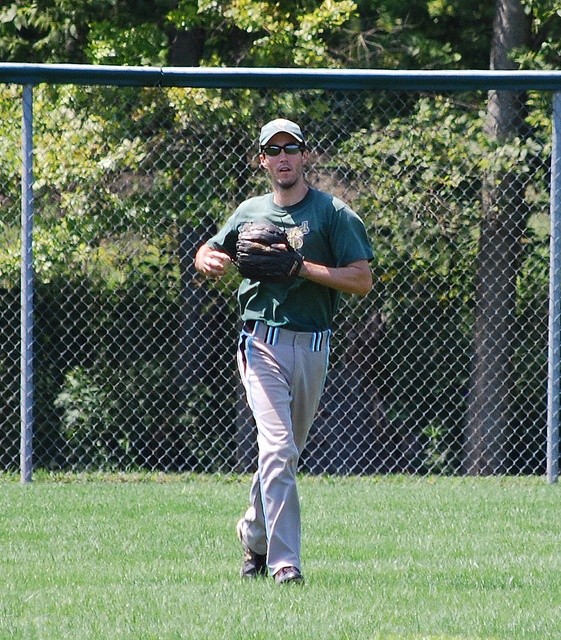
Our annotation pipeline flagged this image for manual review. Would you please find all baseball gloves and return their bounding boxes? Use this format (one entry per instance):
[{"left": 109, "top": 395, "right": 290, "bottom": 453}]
[{"left": 237, "top": 223, "right": 306, "bottom": 283}]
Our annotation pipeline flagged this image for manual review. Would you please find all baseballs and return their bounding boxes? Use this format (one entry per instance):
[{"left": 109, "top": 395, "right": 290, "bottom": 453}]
[{"left": 214, "top": 250, "right": 229, "bottom": 272}]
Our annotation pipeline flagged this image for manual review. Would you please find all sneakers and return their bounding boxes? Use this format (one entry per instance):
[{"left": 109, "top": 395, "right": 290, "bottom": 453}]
[
  {"left": 274, "top": 566, "right": 305, "bottom": 585},
  {"left": 237, "top": 519, "right": 269, "bottom": 580}
]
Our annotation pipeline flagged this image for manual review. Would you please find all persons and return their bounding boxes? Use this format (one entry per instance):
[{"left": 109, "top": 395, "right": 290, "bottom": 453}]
[{"left": 195, "top": 117, "right": 376, "bottom": 584}]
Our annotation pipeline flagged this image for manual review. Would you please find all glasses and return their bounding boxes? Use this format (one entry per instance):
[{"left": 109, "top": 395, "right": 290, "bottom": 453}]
[{"left": 261, "top": 146, "right": 303, "bottom": 156}]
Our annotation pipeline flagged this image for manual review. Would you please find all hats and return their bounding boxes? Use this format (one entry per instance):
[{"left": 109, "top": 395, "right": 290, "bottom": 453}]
[{"left": 257, "top": 119, "right": 305, "bottom": 146}]
